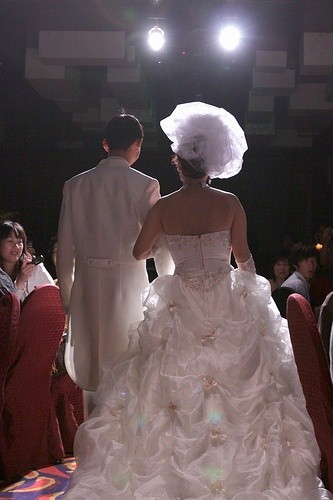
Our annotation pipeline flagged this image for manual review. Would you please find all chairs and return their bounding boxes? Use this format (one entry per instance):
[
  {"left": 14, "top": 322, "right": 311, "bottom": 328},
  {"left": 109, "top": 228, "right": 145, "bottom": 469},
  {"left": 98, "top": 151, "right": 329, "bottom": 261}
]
[
  {"left": 271, "top": 286, "right": 333, "bottom": 493},
  {"left": 0, "top": 284, "right": 85, "bottom": 491}
]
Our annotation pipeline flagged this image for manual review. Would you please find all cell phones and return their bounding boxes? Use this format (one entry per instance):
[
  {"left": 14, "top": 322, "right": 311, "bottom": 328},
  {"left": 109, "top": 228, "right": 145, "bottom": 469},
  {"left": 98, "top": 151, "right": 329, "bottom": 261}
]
[{"left": 25, "top": 255, "right": 44, "bottom": 267}]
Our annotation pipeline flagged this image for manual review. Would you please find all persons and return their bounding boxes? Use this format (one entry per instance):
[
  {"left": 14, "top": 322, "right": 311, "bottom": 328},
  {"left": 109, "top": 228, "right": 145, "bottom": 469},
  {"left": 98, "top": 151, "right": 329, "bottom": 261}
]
[
  {"left": 317, "top": 291, "right": 332, "bottom": 384},
  {"left": 55, "top": 100, "right": 332, "bottom": 500},
  {"left": 280, "top": 242, "right": 319, "bottom": 302},
  {"left": 0, "top": 221, "right": 54, "bottom": 303},
  {"left": 57, "top": 114, "right": 176, "bottom": 391},
  {"left": 268, "top": 252, "right": 292, "bottom": 291}
]
